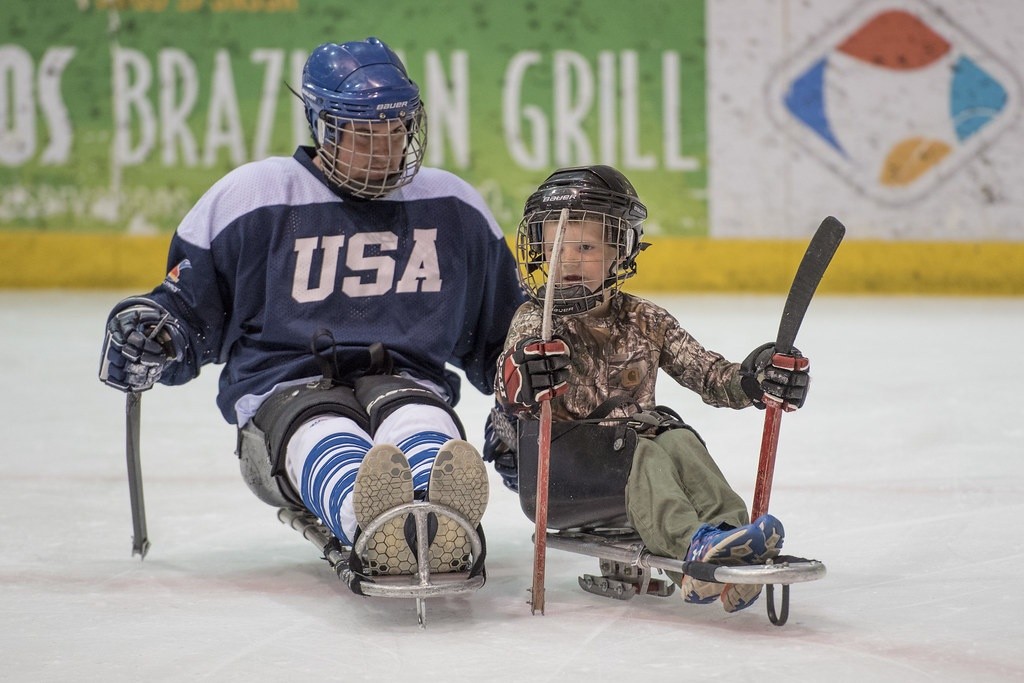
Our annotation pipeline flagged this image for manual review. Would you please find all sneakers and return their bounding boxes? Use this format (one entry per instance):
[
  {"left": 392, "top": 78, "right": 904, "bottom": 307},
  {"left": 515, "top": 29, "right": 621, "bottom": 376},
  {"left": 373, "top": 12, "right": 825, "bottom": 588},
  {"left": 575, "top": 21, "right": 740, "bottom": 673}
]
[
  {"left": 681, "top": 523, "right": 765, "bottom": 604},
  {"left": 424, "top": 439, "right": 489, "bottom": 574},
  {"left": 721, "top": 514, "right": 785, "bottom": 613},
  {"left": 352, "top": 444, "right": 417, "bottom": 574}
]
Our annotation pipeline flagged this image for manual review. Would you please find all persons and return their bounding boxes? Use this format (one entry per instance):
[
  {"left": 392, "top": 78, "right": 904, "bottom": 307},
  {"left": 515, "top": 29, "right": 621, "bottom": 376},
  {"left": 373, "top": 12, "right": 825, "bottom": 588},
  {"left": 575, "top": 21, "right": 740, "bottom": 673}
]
[
  {"left": 96, "top": 35, "right": 529, "bottom": 576},
  {"left": 481, "top": 165, "right": 810, "bottom": 612}
]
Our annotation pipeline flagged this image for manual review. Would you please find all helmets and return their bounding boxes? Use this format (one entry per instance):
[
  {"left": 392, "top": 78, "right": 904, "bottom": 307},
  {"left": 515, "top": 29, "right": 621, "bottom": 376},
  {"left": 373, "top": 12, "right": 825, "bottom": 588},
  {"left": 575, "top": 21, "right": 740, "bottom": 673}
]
[
  {"left": 523, "top": 165, "right": 653, "bottom": 274},
  {"left": 300, "top": 37, "right": 421, "bottom": 143}
]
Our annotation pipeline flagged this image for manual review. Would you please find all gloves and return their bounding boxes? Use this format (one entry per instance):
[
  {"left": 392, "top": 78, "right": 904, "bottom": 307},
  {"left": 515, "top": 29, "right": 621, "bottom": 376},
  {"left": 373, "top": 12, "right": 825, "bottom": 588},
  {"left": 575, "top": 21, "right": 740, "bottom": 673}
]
[
  {"left": 98, "top": 305, "right": 190, "bottom": 393},
  {"left": 482, "top": 404, "right": 521, "bottom": 494},
  {"left": 741, "top": 343, "right": 810, "bottom": 413},
  {"left": 497, "top": 337, "right": 572, "bottom": 414}
]
